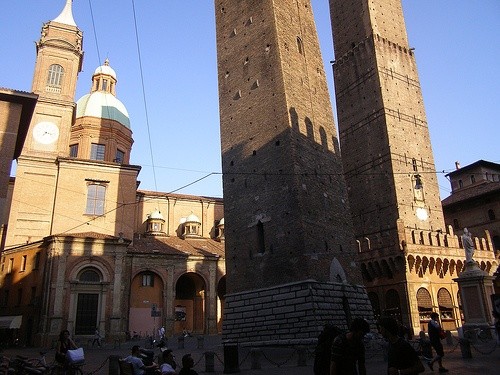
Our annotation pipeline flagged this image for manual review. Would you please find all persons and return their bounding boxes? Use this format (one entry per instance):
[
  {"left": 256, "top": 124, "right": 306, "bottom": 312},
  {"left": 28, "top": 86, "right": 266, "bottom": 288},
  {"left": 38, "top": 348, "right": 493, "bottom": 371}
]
[
  {"left": 461, "top": 226, "right": 478, "bottom": 262},
  {"left": 310, "top": 311, "right": 474, "bottom": 375},
  {"left": 55, "top": 326, "right": 200, "bottom": 375}
]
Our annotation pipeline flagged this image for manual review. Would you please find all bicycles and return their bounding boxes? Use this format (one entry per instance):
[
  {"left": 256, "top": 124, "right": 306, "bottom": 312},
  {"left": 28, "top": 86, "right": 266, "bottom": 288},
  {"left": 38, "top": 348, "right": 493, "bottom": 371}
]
[{"left": 2, "top": 349, "right": 84, "bottom": 375}]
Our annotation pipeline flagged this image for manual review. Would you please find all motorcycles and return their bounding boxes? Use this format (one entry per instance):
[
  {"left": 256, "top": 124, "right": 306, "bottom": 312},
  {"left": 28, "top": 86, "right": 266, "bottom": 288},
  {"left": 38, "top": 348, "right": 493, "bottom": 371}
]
[{"left": 154, "top": 337, "right": 176, "bottom": 370}]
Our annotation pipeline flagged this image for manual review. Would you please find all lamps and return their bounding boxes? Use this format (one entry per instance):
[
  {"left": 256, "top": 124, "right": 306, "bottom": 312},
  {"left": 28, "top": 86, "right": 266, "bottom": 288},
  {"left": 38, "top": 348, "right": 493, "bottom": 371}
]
[
  {"left": 118, "top": 232, "right": 125, "bottom": 244},
  {"left": 415, "top": 174, "right": 424, "bottom": 190}
]
